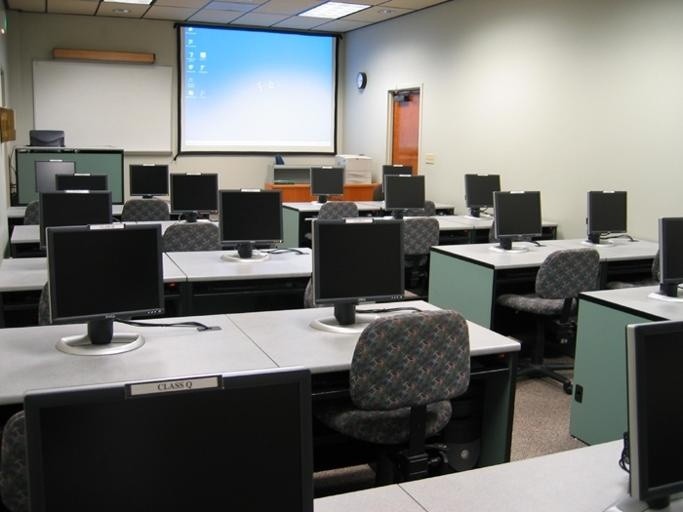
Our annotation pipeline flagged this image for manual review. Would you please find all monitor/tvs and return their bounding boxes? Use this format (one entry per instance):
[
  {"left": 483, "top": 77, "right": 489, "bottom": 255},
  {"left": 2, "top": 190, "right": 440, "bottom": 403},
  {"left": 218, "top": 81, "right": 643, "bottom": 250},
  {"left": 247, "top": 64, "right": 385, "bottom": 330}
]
[
  {"left": 129, "top": 163, "right": 169, "bottom": 199},
  {"left": 25, "top": 368, "right": 313, "bottom": 511},
  {"left": 169, "top": 173, "right": 219, "bottom": 221},
  {"left": 493, "top": 190, "right": 542, "bottom": 252},
  {"left": 218, "top": 187, "right": 284, "bottom": 258},
  {"left": 54, "top": 173, "right": 110, "bottom": 190},
  {"left": 311, "top": 218, "right": 407, "bottom": 334},
  {"left": 45, "top": 223, "right": 165, "bottom": 356},
  {"left": 385, "top": 173, "right": 426, "bottom": 216},
  {"left": 29, "top": 129, "right": 65, "bottom": 146},
  {"left": 39, "top": 191, "right": 112, "bottom": 246},
  {"left": 465, "top": 173, "right": 501, "bottom": 218},
  {"left": 625, "top": 320, "right": 682, "bottom": 509},
  {"left": 382, "top": 164, "right": 414, "bottom": 193},
  {"left": 586, "top": 189, "right": 628, "bottom": 249},
  {"left": 657, "top": 215, "right": 683, "bottom": 296},
  {"left": 310, "top": 166, "right": 345, "bottom": 201}
]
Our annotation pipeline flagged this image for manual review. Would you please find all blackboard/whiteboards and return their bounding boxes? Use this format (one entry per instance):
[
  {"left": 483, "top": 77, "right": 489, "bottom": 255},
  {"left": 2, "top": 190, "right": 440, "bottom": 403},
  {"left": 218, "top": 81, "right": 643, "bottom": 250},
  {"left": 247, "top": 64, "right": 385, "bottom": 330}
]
[{"left": 32, "top": 60, "right": 176, "bottom": 157}]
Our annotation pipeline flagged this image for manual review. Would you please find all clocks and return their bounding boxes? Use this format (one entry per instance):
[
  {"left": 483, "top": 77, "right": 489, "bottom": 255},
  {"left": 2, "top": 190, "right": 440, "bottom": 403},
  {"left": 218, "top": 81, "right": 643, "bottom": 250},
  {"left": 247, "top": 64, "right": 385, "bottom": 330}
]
[{"left": 356, "top": 73, "right": 367, "bottom": 89}]
